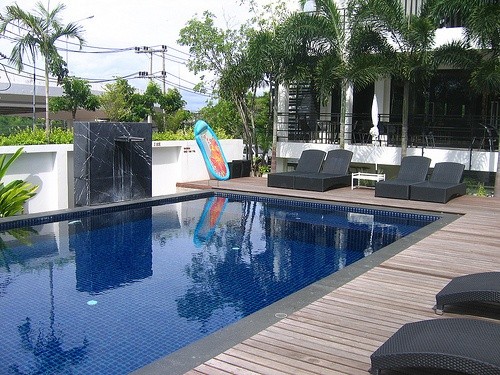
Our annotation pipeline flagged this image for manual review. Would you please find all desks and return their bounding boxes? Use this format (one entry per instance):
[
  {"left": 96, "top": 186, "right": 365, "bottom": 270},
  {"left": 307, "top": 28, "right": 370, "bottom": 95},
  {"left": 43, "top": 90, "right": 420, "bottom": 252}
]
[{"left": 351, "top": 171, "right": 386, "bottom": 190}]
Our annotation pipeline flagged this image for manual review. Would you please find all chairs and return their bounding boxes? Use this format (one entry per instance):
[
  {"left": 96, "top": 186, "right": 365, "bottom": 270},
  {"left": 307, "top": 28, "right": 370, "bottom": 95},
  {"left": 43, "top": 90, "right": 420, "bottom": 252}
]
[
  {"left": 368, "top": 317, "right": 500, "bottom": 375},
  {"left": 375, "top": 155, "right": 432, "bottom": 200},
  {"left": 469, "top": 122, "right": 499, "bottom": 151},
  {"left": 267, "top": 149, "right": 326, "bottom": 189},
  {"left": 435, "top": 271, "right": 500, "bottom": 314},
  {"left": 410, "top": 162, "right": 466, "bottom": 204},
  {"left": 299, "top": 119, "right": 437, "bottom": 148},
  {"left": 294, "top": 149, "right": 353, "bottom": 191}
]
